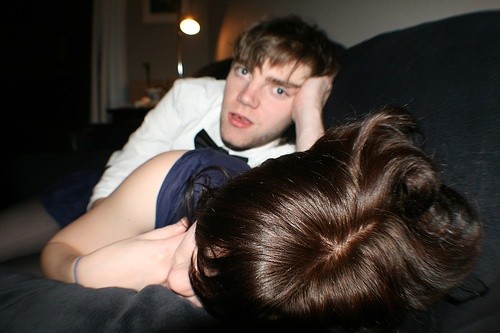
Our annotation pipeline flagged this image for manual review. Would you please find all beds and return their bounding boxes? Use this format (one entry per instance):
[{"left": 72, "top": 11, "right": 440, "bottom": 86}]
[{"left": 0, "top": 9, "right": 500, "bottom": 333}]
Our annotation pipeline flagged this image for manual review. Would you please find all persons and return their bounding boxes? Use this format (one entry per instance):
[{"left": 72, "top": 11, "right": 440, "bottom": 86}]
[
  {"left": 86, "top": 12, "right": 353, "bottom": 214},
  {"left": 1, "top": 102, "right": 482, "bottom": 333}
]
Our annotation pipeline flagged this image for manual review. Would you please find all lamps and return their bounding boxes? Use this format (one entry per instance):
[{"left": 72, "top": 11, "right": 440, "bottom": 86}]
[{"left": 177, "top": 10, "right": 201, "bottom": 79}]
